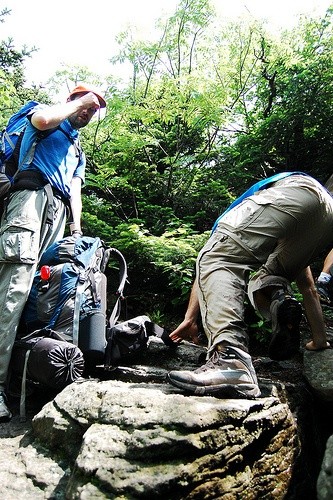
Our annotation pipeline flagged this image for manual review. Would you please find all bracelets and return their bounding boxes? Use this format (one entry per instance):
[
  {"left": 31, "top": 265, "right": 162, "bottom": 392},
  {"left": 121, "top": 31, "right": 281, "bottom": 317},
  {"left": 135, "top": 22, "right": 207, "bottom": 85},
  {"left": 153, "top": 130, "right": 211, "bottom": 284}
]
[{"left": 68, "top": 224, "right": 82, "bottom": 235}]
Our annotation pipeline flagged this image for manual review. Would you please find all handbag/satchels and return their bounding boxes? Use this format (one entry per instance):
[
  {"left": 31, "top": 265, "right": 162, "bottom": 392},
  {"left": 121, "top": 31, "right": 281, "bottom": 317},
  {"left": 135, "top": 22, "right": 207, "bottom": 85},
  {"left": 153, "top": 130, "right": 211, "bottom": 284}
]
[{"left": 104, "top": 315, "right": 173, "bottom": 371}]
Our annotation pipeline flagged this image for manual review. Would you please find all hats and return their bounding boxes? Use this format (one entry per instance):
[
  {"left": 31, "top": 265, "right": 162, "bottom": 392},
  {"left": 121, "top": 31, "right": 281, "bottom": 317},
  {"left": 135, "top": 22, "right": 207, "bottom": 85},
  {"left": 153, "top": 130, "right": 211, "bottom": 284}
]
[{"left": 66, "top": 85, "right": 107, "bottom": 108}]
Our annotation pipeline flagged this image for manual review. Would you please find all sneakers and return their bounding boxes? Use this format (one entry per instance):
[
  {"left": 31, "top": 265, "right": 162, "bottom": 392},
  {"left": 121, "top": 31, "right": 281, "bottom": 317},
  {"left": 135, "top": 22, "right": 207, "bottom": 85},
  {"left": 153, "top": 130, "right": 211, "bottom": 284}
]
[
  {"left": 168, "top": 345, "right": 261, "bottom": 398},
  {"left": 0, "top": 396, "right": 12, "bottom": 418},
  {"left": 269, "top": 290, "right": 302, "bottom": 362},
  {"left": 314, "top": 278, "right": 333, "bottom": 302}
]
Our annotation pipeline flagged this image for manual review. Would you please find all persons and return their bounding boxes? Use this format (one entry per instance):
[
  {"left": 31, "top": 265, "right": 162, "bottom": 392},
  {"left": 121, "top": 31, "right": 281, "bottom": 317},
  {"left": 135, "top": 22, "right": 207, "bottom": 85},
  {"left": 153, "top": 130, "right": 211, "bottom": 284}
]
[
  {"left": 166, "top": 171, "right": 333, "bottom": 396},
  {"left": 0, "top": 85, "right": 106, "bottom": 420}
]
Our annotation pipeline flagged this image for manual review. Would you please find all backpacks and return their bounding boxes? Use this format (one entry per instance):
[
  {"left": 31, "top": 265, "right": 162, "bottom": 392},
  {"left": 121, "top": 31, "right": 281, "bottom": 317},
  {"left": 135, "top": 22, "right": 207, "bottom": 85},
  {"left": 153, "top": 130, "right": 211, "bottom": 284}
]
[
  {"left": 6, "top": 237, "right": 127, "bottom": 422},
  {"left": 0, "top": 101, "right": 82, "bottom": 207}
]
